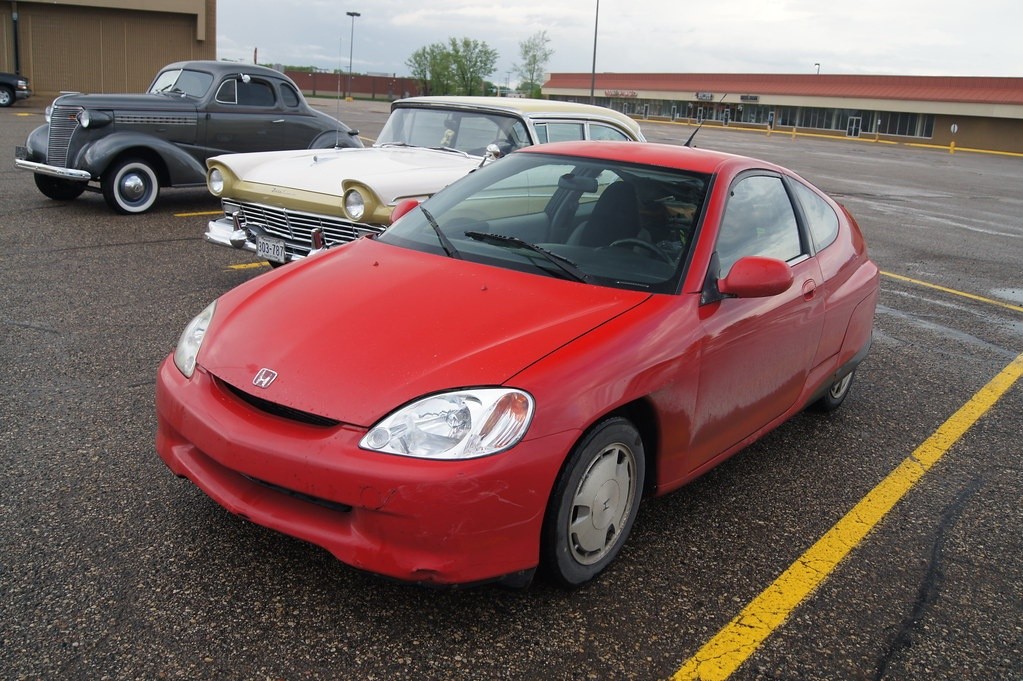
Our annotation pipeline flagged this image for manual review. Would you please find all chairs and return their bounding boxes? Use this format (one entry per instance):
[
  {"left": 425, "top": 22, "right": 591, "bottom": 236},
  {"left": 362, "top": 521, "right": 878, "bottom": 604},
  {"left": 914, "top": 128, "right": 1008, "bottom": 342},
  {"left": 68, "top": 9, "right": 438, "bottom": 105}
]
[{"left": 567, "top": 181, "right": 651, "bottom": 252}]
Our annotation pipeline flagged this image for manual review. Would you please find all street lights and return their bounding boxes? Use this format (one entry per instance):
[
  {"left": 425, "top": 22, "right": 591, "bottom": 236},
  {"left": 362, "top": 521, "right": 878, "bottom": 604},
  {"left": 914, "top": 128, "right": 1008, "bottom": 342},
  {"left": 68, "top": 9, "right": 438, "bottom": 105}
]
[
  {"left": 346, "top": 11, "right": 361, "bottom": 102},
  {"left": 814, "top": 62, "right": 822, "bottom": 75}
]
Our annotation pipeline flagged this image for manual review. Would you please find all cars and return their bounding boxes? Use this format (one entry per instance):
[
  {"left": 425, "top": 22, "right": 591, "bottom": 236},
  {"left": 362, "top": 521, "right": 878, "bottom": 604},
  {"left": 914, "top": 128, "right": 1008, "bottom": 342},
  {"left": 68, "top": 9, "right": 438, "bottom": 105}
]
[
  {"left": 0, "top": 71, "right": 32, "bottom": 109},
  {"left": 203, "top": 94, "right": 651, "bottom": 271},
  {"left": 12, "top": 61, "right": 365, "bottom": 215},
  {"left": 157, "top": 139, "right": 881, "bottom": 591}
]
